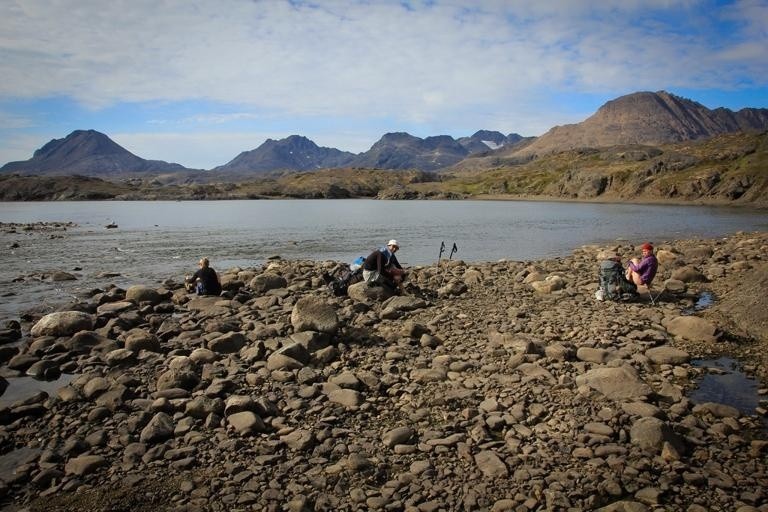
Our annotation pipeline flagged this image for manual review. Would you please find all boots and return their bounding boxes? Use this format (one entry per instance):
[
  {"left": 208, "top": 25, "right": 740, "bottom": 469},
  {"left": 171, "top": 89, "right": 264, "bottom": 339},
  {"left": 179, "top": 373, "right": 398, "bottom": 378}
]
[{"left": 398, "top": 282, "right": 411, "bottom": 296}]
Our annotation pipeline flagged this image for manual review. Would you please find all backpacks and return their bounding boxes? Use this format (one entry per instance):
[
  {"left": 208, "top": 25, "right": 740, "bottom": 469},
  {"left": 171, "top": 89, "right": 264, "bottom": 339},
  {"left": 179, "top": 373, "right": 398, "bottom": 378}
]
[
  {"left": 350, "top": 255, "right": 366, "bottom": 272},
  {"left": 600, "top": 256, "right": 637, "bottom": 301}
]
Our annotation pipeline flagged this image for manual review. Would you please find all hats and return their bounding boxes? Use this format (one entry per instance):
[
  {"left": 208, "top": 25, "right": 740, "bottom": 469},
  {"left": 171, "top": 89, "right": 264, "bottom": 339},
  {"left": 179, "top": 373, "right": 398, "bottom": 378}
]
[
  {"left": 198, "top": 257, "right": 209, "bottom": 265},
  {"left": 387, "top": 239, "right": 400, "bottom": 250},
  {"left": 640, "top": 244, "right": 655, "bottom": 251}
]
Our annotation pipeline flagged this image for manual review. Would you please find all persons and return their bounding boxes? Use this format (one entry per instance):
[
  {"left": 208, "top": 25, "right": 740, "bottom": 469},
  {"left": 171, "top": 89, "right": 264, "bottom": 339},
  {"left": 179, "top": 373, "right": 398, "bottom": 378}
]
[
  {"left": 182, "top": 256, "right": 223, "bottom": 297},
  {"left": 361, "top": 238, "right": 409, "bottom": 296},
  {"left": 625, "top": 243, "right": 659, "bottom": 289}
]
